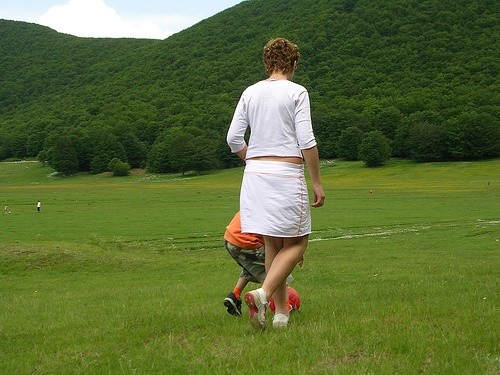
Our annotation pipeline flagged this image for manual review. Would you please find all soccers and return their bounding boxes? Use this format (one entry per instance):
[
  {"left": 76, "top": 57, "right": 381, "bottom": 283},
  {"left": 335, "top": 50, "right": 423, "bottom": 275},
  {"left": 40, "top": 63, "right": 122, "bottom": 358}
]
[{"left": 268, "top": 286, "right": 300, "bottom": 314}]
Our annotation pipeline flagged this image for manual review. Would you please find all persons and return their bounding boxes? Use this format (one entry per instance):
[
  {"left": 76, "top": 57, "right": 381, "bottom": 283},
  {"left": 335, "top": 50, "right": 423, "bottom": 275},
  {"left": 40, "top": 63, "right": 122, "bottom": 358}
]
[
  {"left": 227, "top": 39, "right": 325, "bottom": 330},
  {"left": 4, "top": 200, "right": 41, "bottom": 215},
  {"left": 223, "top": 210, "right": 304, "bottom": 316}
]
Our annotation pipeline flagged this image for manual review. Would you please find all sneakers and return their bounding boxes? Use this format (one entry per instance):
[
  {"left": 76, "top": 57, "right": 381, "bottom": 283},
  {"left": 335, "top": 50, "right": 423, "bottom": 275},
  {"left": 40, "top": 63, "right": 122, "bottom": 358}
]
[
  {"left": 272, "top": 305, "right": 293, "bottom": 327},
  {"left": 224, "top": 293, "right": 242, "bottom": 317},
  {"left": 244, "top": 288, "right": 271, "bottom": 330}
]
